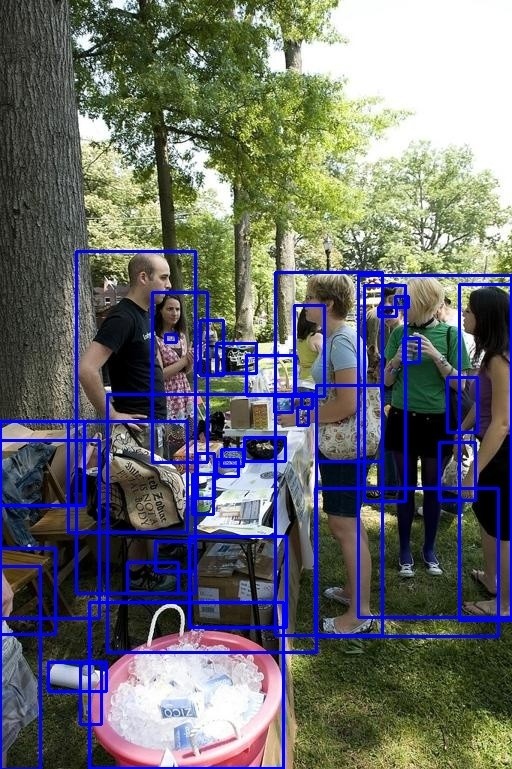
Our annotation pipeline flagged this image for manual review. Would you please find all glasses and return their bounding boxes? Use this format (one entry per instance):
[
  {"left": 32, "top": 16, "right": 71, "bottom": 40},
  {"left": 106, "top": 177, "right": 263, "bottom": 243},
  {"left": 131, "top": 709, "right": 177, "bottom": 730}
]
[
  {"left": 395, "top": 297, "right": 409, "bottom": 307},
  {"left": 378, "top": 307, "right": 396, "bottom": 317}
]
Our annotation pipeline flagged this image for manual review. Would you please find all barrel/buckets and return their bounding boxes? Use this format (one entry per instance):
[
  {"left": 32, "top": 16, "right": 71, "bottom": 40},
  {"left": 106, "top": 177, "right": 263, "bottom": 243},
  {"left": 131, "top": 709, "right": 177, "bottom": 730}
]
[{"left": 89, "top": 602, "right": 284, "bottom": 769}]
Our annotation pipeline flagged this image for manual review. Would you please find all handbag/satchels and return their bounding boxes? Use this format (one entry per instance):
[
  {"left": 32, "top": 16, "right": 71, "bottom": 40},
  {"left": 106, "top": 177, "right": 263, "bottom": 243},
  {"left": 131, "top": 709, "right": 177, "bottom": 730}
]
[
  {"left": 316, "top": 385, "right": 383, "bottom": 461},
  {"left": 447, "top": 377, "right": 478, "bottom": 433},
  {"left": 439, "top": 442, "right": 476, "bottom": 501},
  {"left": 69, "top": 421, "right": 187, "bottom": 532}
]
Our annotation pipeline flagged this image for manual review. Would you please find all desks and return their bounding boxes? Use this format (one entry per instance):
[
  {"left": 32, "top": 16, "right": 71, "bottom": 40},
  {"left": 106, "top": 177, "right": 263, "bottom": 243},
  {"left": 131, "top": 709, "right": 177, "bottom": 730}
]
[
  {"left": 107, "top": 394, "right": 317, "bottom": 652},
  {"left": 216, "top": 344, "right": 256, "bottom": 373}
]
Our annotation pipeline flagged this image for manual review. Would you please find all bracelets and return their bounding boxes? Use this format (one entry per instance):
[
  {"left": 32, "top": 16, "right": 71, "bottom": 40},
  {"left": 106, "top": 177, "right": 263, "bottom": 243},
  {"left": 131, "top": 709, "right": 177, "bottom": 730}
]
[
  {"left": 435, "top": 355, "right": 446, "bottom": 367},
  {"left": 387, "top": 359, "right": 401, "bottom": 375},
  {"left": 297, "top": 407, "right": 309, "bottom": 425},
  {"left": 455, "top": 438, "right": 463, "bottom": 440},
  {"left": 301, "top": 394, "right": 312, "bottom": 408}
]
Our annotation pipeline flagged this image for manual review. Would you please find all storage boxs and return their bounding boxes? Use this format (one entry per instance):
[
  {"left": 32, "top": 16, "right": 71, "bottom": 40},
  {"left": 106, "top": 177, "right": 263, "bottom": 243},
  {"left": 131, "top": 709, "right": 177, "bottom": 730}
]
[
  {"left": 190, "top": 536, "right": 287, "bottom": 628},
  {"left": 0, "top": 421, "right": 85, "bottom": 506}
]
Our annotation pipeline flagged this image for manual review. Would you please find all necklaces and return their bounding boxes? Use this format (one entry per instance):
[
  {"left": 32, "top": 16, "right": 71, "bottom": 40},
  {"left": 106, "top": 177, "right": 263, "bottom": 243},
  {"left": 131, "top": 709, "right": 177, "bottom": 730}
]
[
  {"left": 413, "top": 318, "right": 434, "bottom": 330},
  {"left": 165, "top": 335, "right": 176, "bottom": 342}
]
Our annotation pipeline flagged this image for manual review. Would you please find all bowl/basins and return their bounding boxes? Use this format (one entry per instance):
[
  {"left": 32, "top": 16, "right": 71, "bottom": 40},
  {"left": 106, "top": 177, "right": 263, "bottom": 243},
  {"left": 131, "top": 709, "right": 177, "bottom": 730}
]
[
  {"left": 216, "top": 460, "right": 239, "bottom": 476},
  {"left": 221, "top": 450, "right": 244, "bottom": 466},
  {"left": 245, "top": 437, "right": 286, "bottom": 461}
]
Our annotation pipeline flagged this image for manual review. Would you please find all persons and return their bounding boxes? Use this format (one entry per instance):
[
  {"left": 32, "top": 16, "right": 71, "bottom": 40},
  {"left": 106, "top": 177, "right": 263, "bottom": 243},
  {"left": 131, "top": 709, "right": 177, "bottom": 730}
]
[
  {"left": 76, "top": 251, "right": 196, "bottom": 594},
  {"left": 276, "top": 273, "right": 383, "bottom": 635},
  {"left": 295, "top": 306, "right": 325, "bottom": 396},
  {"left": 152, "top": 293, "right": 207, "bottom": 462},
  {"left": 0, "top": 567, "right": 41, "bottom": 769},
  {"left": 201, "top": 320, "right": 224, "bottom": 375},
  {"left": 358, "top": 276, "right": 484, "bottom": 577},
  {"left": 453, "top": 288, "right": 512, "bottom": 616}
]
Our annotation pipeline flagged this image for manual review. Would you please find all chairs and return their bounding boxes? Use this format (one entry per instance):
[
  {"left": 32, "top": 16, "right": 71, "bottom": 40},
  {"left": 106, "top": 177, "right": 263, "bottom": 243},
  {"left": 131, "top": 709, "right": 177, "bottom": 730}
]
[
  {"left": 0, "top": 548, "right": 56, "bottom": 635},
  {"left": 0, "top": 441, "right": 99, "bottom": 618}
]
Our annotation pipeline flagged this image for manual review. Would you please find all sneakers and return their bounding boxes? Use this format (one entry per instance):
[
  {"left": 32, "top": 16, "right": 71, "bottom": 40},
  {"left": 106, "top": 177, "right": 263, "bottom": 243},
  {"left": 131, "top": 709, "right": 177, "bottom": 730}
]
[
  {"left": 156, "top": 541, "right": 196, "bottom": 571},
  {"left": 127, "top": 563, "right": 178, "bottom": 593},
  {"left": 316, "top": 489, "right": 498, "bottom": 637}
]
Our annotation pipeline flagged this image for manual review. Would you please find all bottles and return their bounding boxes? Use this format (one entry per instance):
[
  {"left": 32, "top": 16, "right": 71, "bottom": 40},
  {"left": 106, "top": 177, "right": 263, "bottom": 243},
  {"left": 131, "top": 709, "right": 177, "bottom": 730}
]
[
  {"left": 192, "top": 475, "right": 213, "bottom": 514},
  {"left": 80, "top": 663, "right": 101, "bottom": 724},
  {"left": 198, "top": 453, "right": 214, "bottom": 499}
]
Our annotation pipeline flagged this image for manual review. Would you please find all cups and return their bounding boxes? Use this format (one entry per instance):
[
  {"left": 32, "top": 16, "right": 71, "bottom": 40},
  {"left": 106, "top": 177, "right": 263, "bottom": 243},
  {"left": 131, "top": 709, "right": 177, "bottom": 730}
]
[
  {"left": 275, "top": 396, "right": 292, "bottom": 413},
  {"left": 404, "top": 339, "right": 419, "bottom": 363}
]
